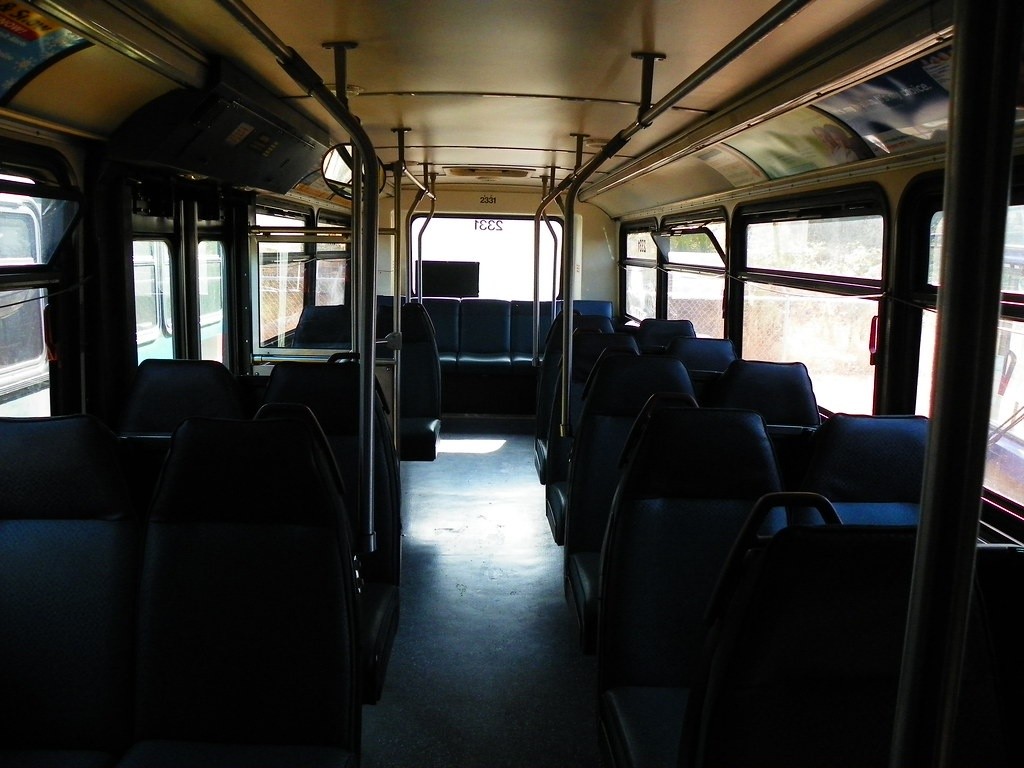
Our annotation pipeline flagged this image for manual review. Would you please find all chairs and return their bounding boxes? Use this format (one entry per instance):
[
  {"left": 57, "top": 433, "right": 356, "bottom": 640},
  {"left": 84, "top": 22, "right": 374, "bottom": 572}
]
[{"left": 1, "top": 294, "right": 1024, "bottom": 768}]
